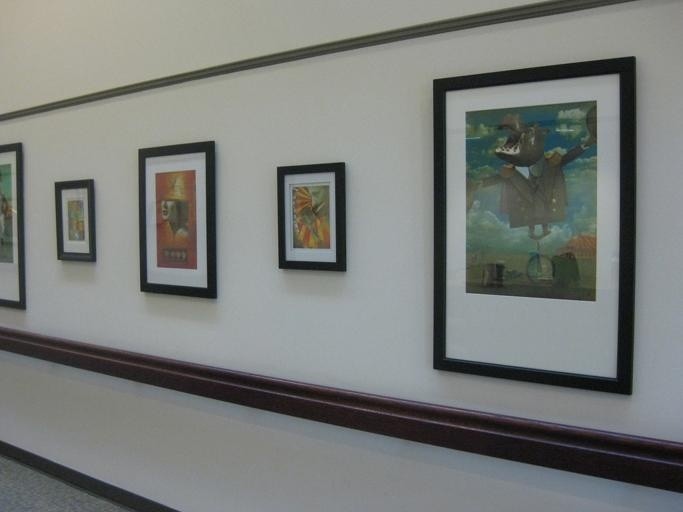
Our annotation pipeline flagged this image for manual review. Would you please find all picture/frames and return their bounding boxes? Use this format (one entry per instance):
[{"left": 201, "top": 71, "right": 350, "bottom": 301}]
[
  {"left": 55, "top": 179, "right": 96, "bottom": 263},
  {"left": 0, "top": 142, "right": 26, "bottom": 310},
  {"left": 276, "top": 162, "right": 347, "bottom": 271},
  {"left": 433, "top": 55, "right": 636, "bottom": 395},
  {"left": 137, "top": 141, "right": 217, "bottom": 297}
]
